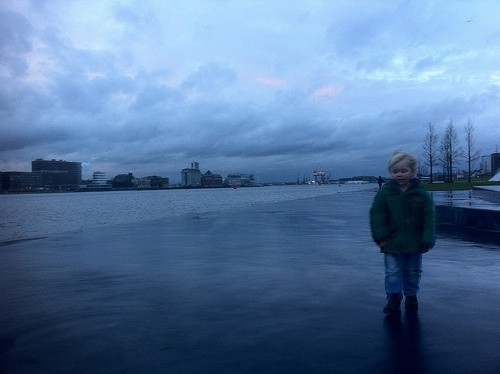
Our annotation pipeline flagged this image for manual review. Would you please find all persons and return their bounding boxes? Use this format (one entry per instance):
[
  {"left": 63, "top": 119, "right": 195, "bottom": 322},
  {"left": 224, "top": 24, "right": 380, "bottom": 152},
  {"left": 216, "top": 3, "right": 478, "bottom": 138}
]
[
  {"left": 378, "top": 176, "right": 383, "bottom": 189},
  {"left": 369, "top": 153, "right": 437, "bottom": 315}
]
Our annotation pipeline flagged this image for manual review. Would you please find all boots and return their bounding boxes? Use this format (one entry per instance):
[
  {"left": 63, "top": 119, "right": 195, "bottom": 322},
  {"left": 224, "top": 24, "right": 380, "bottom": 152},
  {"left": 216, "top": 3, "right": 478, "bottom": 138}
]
[
  {"left": 404, "top": 292, "right": 419, "bottom": 316},
  {"left": 383, "top": 292, "right": 402, "bottom": 323}
]
